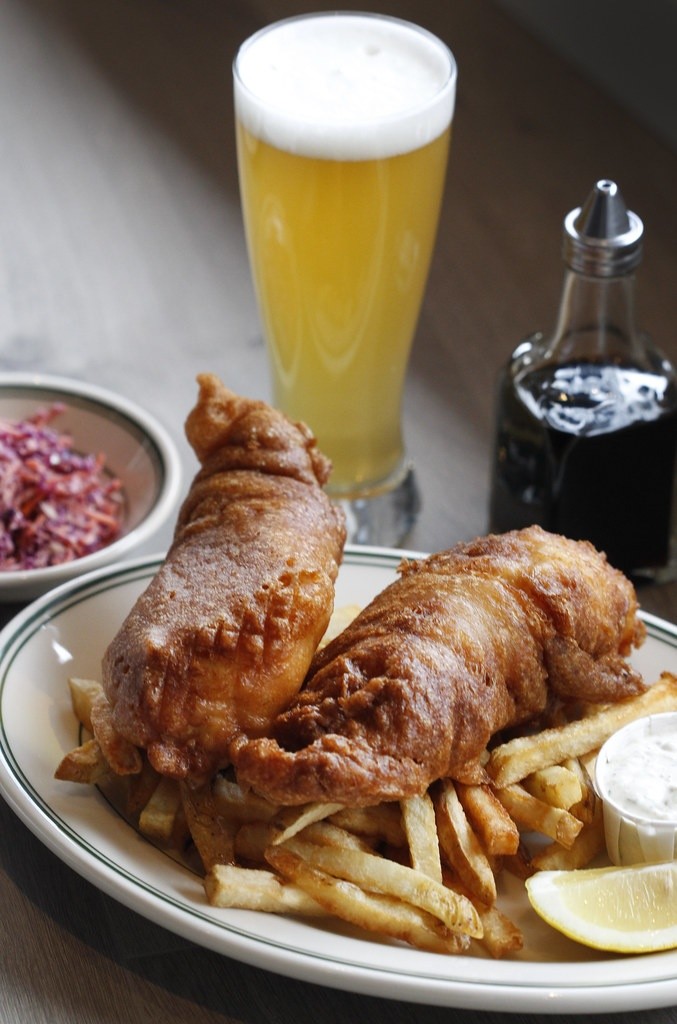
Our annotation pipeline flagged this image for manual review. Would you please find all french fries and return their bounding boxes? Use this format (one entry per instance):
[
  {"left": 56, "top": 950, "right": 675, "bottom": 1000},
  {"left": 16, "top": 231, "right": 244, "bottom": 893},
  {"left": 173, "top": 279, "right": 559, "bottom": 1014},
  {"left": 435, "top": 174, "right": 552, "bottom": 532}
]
[{"left": 53, "top": 605, "right": 677, "bottom": 961}]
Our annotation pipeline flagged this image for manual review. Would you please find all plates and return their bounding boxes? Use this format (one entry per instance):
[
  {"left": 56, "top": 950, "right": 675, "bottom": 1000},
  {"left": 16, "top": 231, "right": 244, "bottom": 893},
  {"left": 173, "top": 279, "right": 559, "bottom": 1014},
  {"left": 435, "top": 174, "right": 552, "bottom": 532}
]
[{"left": 0, "top": 542, "right": 677, "bottom": 1014}]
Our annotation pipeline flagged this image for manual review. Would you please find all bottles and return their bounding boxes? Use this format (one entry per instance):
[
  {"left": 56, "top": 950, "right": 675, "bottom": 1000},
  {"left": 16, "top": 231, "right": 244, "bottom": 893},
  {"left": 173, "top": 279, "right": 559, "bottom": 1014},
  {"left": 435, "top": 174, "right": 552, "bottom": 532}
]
[{"left": 487, "top": 180, "right": 676, "bottom": 591}]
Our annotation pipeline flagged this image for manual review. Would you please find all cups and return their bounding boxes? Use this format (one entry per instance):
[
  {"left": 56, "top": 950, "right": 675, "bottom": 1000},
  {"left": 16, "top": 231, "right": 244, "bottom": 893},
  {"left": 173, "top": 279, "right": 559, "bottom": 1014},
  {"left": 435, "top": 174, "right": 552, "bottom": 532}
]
[{"left": 231, "top": 11, "right": 458, "bottom": 550}]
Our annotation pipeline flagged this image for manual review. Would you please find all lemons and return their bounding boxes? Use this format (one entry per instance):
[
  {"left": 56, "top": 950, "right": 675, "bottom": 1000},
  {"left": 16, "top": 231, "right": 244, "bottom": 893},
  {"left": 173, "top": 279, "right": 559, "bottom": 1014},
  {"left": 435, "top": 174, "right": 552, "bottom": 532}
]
[{"left": 524, "top": 860, "right": 677, "bottom": 953}]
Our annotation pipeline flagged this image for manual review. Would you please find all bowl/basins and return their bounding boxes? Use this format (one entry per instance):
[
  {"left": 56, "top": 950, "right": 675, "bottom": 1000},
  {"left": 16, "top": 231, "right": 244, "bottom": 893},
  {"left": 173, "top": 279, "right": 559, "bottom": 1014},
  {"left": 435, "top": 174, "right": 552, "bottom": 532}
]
[
  {"left": 592, "top": 710, "right": 675, "bottom": 864},
  {"left": 0, "top": 372, "right": 183, "bottom": 603}
]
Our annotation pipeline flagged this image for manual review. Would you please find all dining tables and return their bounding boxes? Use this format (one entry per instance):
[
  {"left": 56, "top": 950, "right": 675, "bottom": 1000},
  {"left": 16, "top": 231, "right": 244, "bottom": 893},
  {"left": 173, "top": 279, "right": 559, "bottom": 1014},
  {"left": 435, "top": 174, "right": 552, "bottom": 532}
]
[{"left": 0, "top": 0, "right": 677, "bottom": 1024}]
